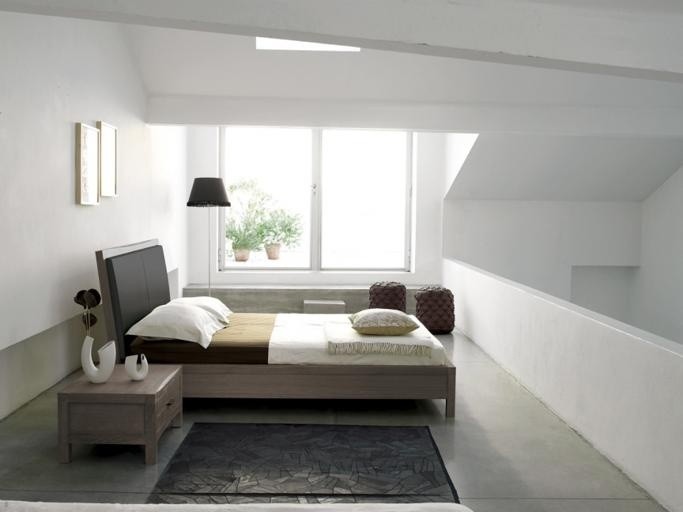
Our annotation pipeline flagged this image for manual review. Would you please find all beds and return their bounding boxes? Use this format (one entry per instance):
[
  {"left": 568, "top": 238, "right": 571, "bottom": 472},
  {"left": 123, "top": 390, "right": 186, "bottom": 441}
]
[{"left": 94, "top": 237, "right": 456, "bottom": 421}]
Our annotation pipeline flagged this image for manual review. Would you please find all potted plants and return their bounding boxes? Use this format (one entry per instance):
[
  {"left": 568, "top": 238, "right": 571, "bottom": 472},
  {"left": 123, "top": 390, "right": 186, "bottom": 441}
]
[
  {"left": 256, "top": 208, "right": 304, "bottom": 260},
  {"left": 226, "top": 181, "right": 290, "bottom": 262}
]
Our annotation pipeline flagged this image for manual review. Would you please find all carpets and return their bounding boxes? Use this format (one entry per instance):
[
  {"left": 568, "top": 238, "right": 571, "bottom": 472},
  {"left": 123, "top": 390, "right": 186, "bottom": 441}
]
[{"left": 143, "top": 421, "right": 458, "bottom": 503}]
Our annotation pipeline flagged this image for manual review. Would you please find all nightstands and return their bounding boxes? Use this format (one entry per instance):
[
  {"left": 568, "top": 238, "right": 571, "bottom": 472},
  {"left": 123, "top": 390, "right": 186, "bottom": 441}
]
[{"left": 57, "top": 363, "right": 183, "bottom": 463}]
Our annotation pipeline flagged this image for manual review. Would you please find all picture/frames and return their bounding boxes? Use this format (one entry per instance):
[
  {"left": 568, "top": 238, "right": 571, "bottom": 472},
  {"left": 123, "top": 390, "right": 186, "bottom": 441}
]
[
  {"left": 75, "top": 122, "right": 100, "bottom": 207},
  {"left": 100, "top": 120, "right": 119, "bottom": 199}
]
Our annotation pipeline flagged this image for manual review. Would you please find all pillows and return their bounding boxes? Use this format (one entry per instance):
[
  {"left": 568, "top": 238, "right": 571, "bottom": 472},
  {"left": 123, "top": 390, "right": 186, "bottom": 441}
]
[
  {"left": 166, "top": 296, "right": 233, "bottom": 324},
  {"left": 348, "top": 308, "right": 420, "bottom": 335},
  {"left": 124, "top": 304, "right": 224, "bottom": 349}
]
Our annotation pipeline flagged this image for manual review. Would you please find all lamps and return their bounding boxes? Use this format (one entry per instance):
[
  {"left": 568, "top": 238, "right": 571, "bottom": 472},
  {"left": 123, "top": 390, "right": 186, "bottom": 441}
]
[{"left": 187, "top": 178, "right": 231, "bottom": 295}]
